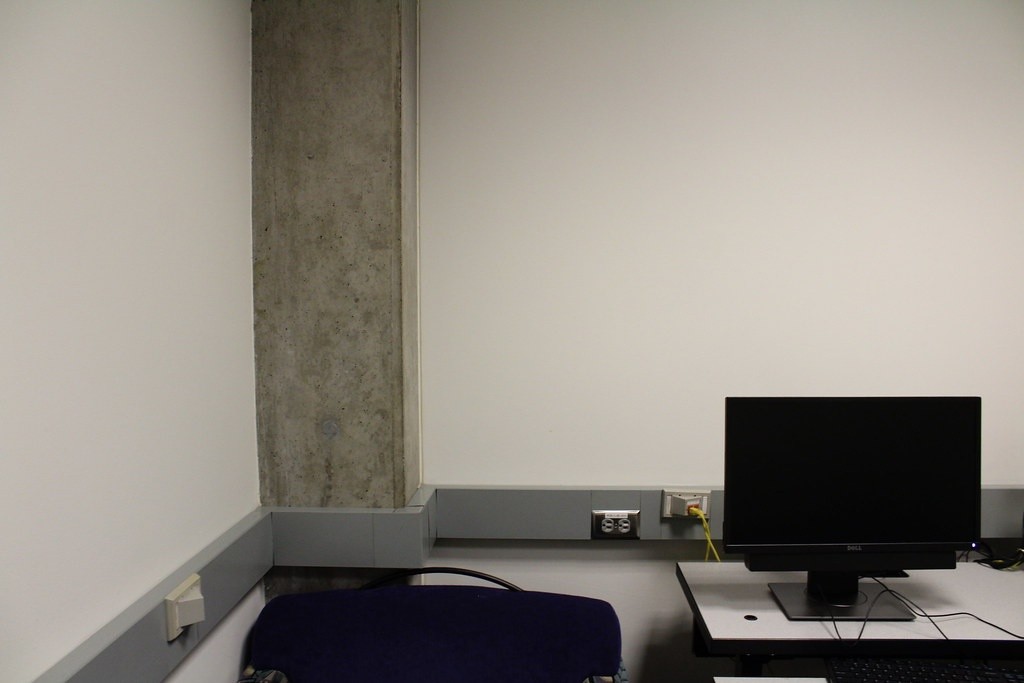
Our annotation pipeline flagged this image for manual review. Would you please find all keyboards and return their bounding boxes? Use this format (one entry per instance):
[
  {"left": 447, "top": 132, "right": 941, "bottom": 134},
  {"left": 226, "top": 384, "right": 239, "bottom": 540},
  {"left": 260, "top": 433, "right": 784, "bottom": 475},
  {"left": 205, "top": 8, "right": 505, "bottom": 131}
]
[{"left": 825, "top": 655, "right": 1024, "bottom": 683}]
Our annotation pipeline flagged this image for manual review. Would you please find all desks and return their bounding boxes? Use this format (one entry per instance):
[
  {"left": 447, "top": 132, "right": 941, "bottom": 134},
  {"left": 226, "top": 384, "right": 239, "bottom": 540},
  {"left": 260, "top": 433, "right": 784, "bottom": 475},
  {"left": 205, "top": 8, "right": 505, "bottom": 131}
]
[{"left": 671, "top": 553, "right": 1024, "bottom": 683}]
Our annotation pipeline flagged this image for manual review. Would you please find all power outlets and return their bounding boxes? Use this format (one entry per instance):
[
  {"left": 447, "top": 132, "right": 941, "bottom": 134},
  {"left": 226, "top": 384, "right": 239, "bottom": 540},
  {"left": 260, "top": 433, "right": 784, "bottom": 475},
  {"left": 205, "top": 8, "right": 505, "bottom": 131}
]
[
  {"left": 659, "top": 488, "right": 711, "bottom": 522},
  {"left": 589, "top": 509, "right": 643, "bottom": 539}
]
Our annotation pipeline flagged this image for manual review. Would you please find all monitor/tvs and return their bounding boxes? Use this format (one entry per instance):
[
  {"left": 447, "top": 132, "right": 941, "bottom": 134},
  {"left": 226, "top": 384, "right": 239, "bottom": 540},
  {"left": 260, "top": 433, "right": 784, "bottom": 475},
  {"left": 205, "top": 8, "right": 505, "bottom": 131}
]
[{"left": 722, "top": 395, "right": 982, "bottom": 554}]
